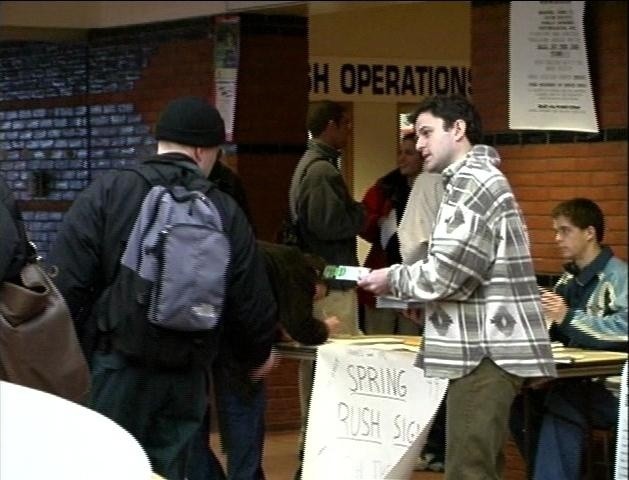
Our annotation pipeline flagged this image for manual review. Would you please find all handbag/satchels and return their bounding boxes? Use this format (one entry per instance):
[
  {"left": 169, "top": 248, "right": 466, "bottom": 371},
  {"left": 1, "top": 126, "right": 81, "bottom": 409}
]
[
  {"left": 275, "top": 222, "right": 316, "bottom": 255},
  {"left": 0, "top": 260, "right": 91, "bottom": 403}
]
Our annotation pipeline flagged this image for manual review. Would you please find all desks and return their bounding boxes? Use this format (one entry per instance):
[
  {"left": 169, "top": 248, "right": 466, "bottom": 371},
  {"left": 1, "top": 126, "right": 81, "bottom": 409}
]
[{"left": 273, "top": 332, "right": 628, "bottom": 480}]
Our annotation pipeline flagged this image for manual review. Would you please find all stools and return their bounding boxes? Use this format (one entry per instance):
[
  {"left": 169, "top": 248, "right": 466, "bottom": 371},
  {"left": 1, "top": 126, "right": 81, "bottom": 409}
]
[{"left": 586, "top": 425, "right": 616, "bottom": 479}]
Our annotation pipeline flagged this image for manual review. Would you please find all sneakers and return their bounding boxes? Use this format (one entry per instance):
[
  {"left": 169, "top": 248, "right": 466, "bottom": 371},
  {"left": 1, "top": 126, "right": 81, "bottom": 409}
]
[{"left": 415, "top": 452, "right": 444, "bottom": 473}]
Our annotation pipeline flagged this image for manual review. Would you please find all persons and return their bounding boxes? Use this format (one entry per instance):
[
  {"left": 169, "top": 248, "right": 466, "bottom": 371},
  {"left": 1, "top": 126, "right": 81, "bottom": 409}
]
[
  {"left": 1, "top": 96, "right": 277, "bottom": 479},
  {"left": 209, "top": 239, "right": 340, "bottom": 479},
  {"left": 289, "top": 95, "right": 628, "bottom": 479}
]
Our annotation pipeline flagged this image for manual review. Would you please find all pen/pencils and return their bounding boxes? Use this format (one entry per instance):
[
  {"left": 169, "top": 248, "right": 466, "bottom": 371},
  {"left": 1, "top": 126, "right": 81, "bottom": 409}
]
[{"left": 321, "top": 308, "right": 330, "bottom": 320}]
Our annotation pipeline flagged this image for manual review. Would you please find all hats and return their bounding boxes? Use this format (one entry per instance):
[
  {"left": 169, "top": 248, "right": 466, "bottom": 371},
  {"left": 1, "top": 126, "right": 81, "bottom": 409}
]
[{"left": 153, "top": 97, "right": 225, "bottom": 147}]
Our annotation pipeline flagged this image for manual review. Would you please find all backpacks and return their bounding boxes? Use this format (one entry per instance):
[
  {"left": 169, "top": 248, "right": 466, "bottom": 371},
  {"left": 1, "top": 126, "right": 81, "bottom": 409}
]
[{"left": 113, "top": 165, "right": 230, "bottom": 362}]
